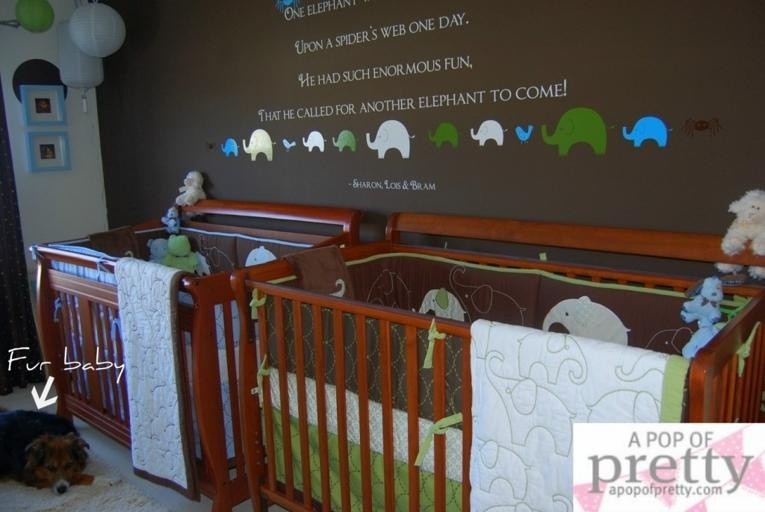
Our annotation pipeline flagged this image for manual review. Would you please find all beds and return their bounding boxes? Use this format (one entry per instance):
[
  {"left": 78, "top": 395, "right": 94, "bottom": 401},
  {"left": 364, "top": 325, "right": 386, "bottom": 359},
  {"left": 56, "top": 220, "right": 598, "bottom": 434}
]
[
  {"left": 27, "top": 199, "right": 363, "bottom": 512},
  {"left": 230, "top": 213, "right": 764, "bottom": 512}
]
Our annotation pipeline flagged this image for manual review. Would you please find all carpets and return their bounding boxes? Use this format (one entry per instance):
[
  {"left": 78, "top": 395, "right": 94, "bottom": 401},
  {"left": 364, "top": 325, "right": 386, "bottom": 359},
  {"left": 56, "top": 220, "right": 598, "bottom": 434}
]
[{"left": 0, "top": 448, "right": 171, "bottom": 512}]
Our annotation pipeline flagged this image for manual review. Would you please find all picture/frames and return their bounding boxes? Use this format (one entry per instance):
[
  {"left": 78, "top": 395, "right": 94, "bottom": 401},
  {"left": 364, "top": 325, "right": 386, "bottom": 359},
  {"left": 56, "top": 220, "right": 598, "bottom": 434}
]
[
  {"left": 24, "top": 130, "right": 72, "bottom": 172},
  {"left": 20, "top": 85, "right": 66, "bottom": 127}
]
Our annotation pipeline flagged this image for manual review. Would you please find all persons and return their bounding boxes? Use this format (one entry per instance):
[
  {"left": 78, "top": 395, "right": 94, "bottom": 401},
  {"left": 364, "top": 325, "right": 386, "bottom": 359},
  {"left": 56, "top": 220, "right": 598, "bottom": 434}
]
[
  {"left": 40, "top": 143, "right": 56, "bottom": 158},
  {"left": 37, "top": 99, "right": 50, "bottom": 113}
]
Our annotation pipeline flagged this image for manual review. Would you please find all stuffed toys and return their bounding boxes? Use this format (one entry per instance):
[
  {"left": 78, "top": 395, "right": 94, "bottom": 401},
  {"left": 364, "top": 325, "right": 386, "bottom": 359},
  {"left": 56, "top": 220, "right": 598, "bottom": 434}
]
[
  {"left": 681, "top": 276, "right": 725, "bottom": 322},
  {"left": 716, "top": 189, "right": 765, "bottom": 281},
  {"left": 159, "top": 234, "right": 200, "bottom": 273},
  {"left": 160, "top": 206, "right": 180, "bottom": 234},
  {"left": 176, "top": 168, "right": 205, "bottom": 207},
  {"left": 146, "top": 238, "right": 168, "bottom": 266}
]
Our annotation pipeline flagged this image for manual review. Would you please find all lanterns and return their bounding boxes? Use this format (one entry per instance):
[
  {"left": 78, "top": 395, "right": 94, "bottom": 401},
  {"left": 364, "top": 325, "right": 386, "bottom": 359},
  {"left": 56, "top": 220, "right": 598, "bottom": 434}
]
[
  {"left": 57, "top": 19, "right": 105, "bottom": 98},
  {"left": 68, "top": 4, "right": 124, "bottom": 58},
  {"left": 15, "top": 0, "right": 54, "bottom": 36}
]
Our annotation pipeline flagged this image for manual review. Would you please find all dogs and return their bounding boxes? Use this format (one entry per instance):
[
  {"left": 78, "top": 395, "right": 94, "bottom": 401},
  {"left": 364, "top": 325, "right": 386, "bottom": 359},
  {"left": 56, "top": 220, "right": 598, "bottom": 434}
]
[{"left": 0, "top": 408, "right": 95, "bottom": 497}]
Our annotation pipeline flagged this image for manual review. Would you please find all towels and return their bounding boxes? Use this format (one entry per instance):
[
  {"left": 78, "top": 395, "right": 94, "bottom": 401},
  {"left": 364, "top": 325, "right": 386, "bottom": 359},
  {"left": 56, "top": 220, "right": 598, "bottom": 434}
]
[
  {"left": 112, "top": 255, "right": 201, "bottom": 502},
  {"left": 465, "top": 313, "right": 688, "bottom": 510}
]
